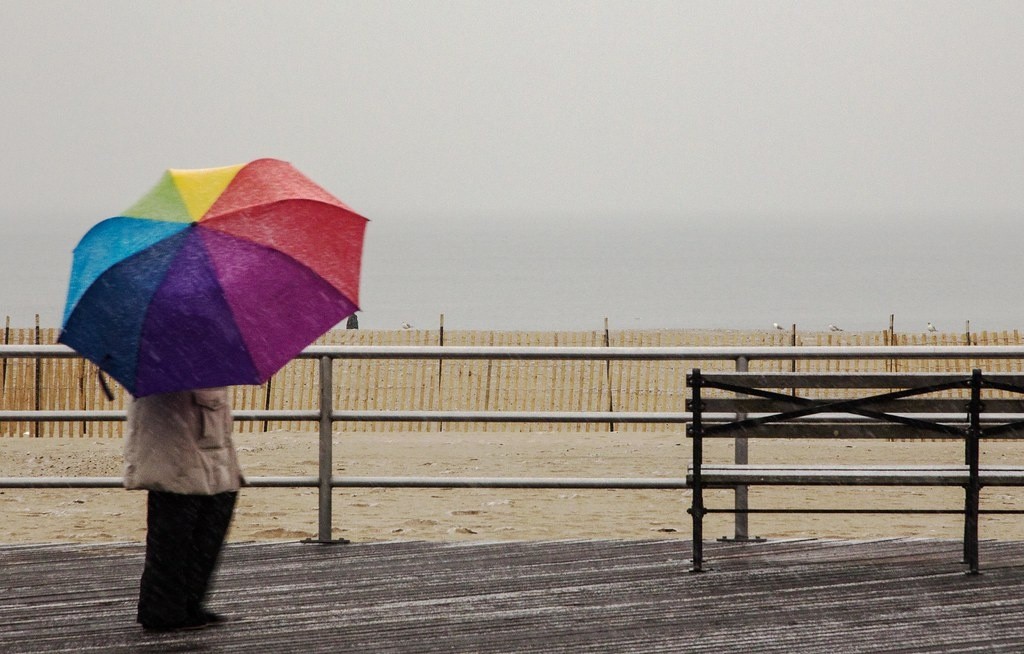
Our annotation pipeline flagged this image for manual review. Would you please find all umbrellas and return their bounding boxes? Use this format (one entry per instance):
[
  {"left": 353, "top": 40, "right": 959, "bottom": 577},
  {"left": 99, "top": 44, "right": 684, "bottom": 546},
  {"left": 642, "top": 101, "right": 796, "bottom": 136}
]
[{"left": 57, "top": 158, "right": 371, "bottom": 401}]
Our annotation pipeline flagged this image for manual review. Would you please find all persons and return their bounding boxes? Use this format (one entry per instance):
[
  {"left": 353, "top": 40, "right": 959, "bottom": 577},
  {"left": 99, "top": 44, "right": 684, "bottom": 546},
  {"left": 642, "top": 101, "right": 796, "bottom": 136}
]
[{"left": 123, "top": 389, "right": 249, "bottom": 631}]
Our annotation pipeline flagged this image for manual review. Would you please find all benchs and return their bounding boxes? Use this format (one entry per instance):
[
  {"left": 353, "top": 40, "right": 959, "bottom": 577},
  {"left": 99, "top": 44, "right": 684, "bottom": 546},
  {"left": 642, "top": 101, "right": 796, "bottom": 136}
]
[{"left": 684, "top": 367, "right": 1024, "bottom": 573}]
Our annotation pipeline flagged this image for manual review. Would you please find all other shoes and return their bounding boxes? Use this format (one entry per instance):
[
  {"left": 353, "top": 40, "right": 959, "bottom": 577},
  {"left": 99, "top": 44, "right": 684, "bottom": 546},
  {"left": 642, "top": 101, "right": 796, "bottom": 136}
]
[{"left": 143, "top": 610, "right": 228, "bottom": 632}]
